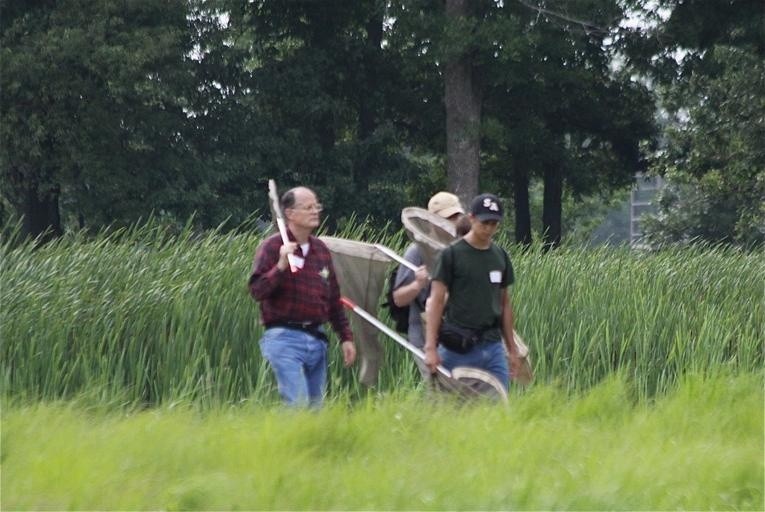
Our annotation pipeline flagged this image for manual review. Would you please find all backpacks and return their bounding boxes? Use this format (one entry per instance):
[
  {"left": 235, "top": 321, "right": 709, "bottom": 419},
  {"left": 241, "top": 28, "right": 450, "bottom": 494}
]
[{"left": 380, "top": 264, "right": 410, "bottom": 336}]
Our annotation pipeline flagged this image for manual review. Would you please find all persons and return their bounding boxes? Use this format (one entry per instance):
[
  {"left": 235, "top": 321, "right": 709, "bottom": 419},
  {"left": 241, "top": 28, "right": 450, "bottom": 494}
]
[
  {"left": 456, "top": 216, "right": 471, "bottom": 238},
  {"left": 422, "top": 193, "right": 518, "bottom": 408},
  {"left": 390, "top": 190, "right": 466, "bottom": 391},
  {"left": 247, "top": 185, "right": 357, "bottom": 411}
]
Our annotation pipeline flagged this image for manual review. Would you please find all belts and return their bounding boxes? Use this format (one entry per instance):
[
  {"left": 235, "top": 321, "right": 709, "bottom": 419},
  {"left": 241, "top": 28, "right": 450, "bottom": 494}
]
[{"left": 263, "top": 320, "right": 330, "bottom": 343}]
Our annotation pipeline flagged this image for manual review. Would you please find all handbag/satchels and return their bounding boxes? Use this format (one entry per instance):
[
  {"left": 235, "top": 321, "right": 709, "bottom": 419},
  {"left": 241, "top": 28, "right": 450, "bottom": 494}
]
[{"left": 440, "top": 323, "right": 477, "bottom": 354}]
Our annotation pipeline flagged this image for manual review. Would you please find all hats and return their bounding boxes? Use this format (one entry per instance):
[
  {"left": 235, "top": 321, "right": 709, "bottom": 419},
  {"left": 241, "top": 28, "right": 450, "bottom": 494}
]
[
  {"left": 428, "top": 191, "right": 465, "bottom": 220},
  {"left": 470, "top": 193, "right": 504, "bottom": 223}
]
